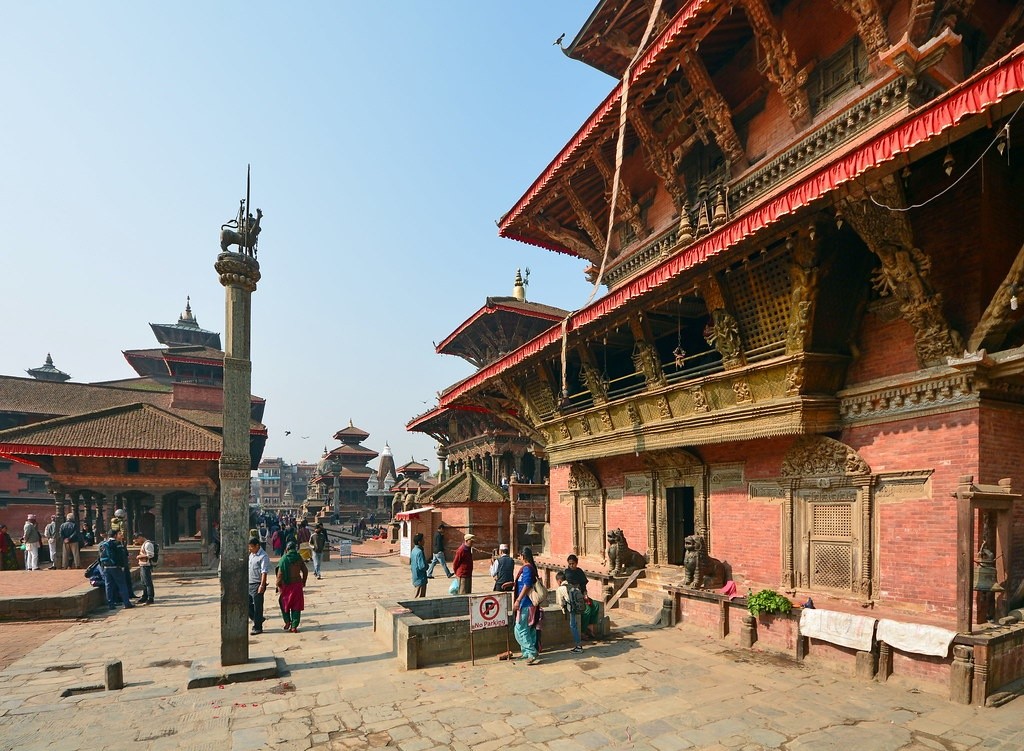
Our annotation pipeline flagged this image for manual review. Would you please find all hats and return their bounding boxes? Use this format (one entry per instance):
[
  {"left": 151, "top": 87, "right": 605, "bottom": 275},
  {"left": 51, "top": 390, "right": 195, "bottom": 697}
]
[
  {"left": 464, "top": 534, "right": 476, "bottom": 541},
  {"left": 27, "top": 514, "right": 36, "bottom": 519},
  {"left": 500, "top": 543, "right": 511, "bottom": 550}
]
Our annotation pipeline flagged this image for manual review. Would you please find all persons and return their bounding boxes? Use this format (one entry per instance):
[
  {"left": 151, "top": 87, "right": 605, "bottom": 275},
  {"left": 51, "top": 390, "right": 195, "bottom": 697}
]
[
  {"left": 20, "top": 514, "right": 42, "bottom": 570},
  {"left": 359, "top": 516, "right": 367, "bottom": 539},
  {"left": 59, "top": 513, "right": 81, "bottom": 570},
  {"left": 411, "top": 533, "right": 430, "bottom": 597},
  {"left": 131, "top": 532, "right": 160, "bottom": 604},
  {"left": 491, "top": 544, "right": 514, "bottom": 592},
  {"left": 257, "top": 510, "right": 313, "bottom": 563},
  {"left": 555, "top": 570, "right": 586, "bottom": 652},
  {"left": 44, "top": 515, "right": 62, "bottom": 570},
  {"left": 97, "top": 529, "right": 140, "bottom": 610},
  {"left": 0, "top": 524, "right": 17, "bottom": 571},
  {"left": 216, "top": 523, "right": 222, "bottom": 578},
  {"left": 309, "top": 525, "right": 325, "bottom": 578},
  {"left": 110, "top": 509, "right": 127, "bottom": 547},
  {"left": 426, "top": 524, "right": 455, "bottom": 579},
  {"left": 452, "top": 534, "right": 477, "bottom": 596},
  {"left": 66, "top": 520, "right": 94, "bottom": 570},
  {"left": 275, "top": 541, "right": 309, "bottom": 633},
  {"left": 564, "top": 555, "right": 600, "bottom": 641},
  {"left": 370, "top": 513, "right": 375, "bottom": 527},
  {"left": 248, "top": 537, "right": 269, "bottom": 635},
  {"left": 502, "top": 545, "right": 547, "bottom": 664}
]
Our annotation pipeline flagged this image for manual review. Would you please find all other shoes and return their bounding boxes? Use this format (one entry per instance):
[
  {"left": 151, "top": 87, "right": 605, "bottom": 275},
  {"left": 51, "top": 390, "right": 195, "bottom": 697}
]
[
  {"left": 290, "top": 628, "right": 298, "bottom": 633},
  {"left": 128, "top": 594, "right": 140, "bottom": 599},
  {"left": 449, "top": 573, "right": 455, "bottom": 578},
  {"left": 113, "top": 601, "right": 122, "bottom": 606},
  {"left": 427, "top": 576, "right": 434, "bottom": 579},
  {"left": 254, "top": 617, "right": 266, "bottom": 627},
  {"left": 283, "top": 621, "right": 291, "bottom": 629},
  {"left": 526, "top": 655, "right": 543, "bottom": 664},
  {"left": 537, "top": 643, "right": 542, "bottom": 652},
  {"left": 251, "top": 628, "right": 263, "bottom": 634},
  {"left": 108, "top": 604, "right": 116, "bottom": 610},
  {"left": 570, "top": 646, "right": 583, "bottom": 653},
  {"left": 585, "top": 628, "right": 596, "bottom": 638},
  {"left": 315, "top": 573, "right": 321, "bottom": 579},
  {"left": 26, "top": 564, "right": 81, "bottom": 571},
  {"left": 143, "top": 600, "right": 155, "bottom": 604},
  {"left": 135, "top": 598, "right": 147, "bottom": 604},
  {"left": 124, "top": 603, "right": 135, "bottom": 608}
]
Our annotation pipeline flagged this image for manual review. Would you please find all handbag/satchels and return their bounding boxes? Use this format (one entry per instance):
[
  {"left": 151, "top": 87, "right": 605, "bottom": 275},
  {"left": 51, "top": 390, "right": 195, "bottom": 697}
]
[
  {"left": 526, "top": 577, "right": 549, "bottom": 607},
  {"left": 449, "top": 577, "right": 459, "bottom": 594}
]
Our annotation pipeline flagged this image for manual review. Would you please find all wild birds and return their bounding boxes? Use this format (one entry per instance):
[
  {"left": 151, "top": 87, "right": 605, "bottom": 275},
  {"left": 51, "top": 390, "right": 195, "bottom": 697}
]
[
  {"left": 420, "top": 400, "right": 428, "bottom": 404},
  {"left": 301, "top": 437, "right": 309, "bottom": 439},
  {"left": 285, "top": 431, "right": 291, "bottom": 436}
]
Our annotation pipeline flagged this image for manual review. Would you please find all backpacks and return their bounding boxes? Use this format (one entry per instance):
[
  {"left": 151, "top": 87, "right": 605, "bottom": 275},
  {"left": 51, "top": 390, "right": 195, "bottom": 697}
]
[
  {"left": 99, "top": 541, "right": 123, "bottom": 566},
  {"left": 143, "top": 541, "right": 160, "bottom": 562},
  {"left": 84, "top": 560, "right": 100, "bottom": 579},
  {"left": 562, "top": 583, "right": 586, "bottom": 614}
]
